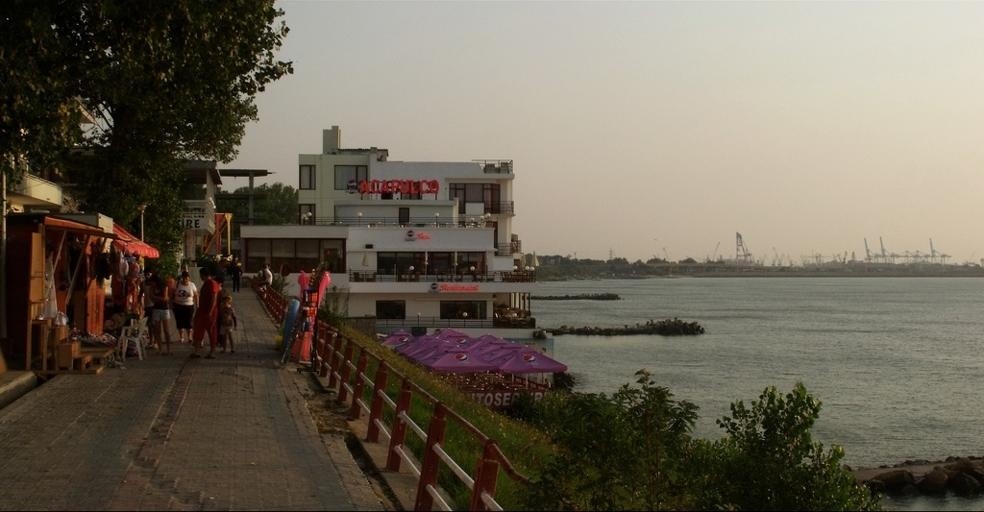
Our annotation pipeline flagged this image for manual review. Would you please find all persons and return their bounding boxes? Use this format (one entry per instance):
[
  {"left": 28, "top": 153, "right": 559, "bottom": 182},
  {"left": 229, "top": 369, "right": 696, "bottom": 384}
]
[{"left": 133, "top": 256, "right": 275, "bottom": 359}]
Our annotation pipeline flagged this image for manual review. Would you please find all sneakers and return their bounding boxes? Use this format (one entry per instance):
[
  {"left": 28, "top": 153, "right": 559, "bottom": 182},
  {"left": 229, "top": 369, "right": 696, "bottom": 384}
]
[
  {"left": 178, "top": 336, "right": 235, "bottom": 358},
  {"left": 146, "top": 344, "right": 158, "bottom": 350}
]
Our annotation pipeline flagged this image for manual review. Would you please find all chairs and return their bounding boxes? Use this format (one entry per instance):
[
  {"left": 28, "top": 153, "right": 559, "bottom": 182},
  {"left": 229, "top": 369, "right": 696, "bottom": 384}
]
[{"left": 114, "top": 317, "right": 151, "bottom": 361}]
